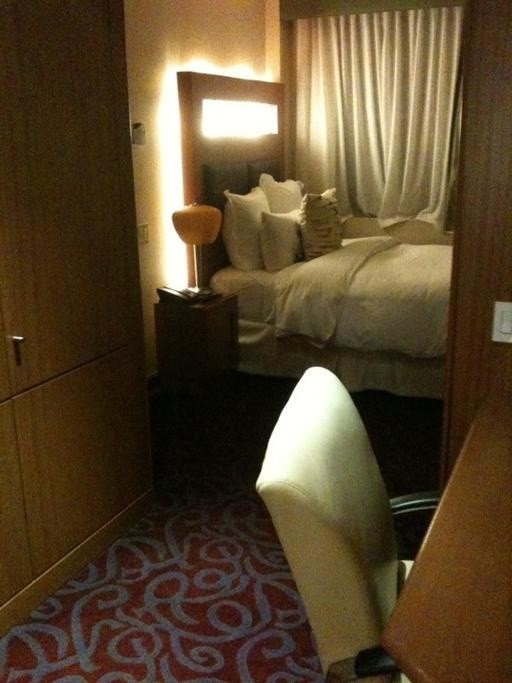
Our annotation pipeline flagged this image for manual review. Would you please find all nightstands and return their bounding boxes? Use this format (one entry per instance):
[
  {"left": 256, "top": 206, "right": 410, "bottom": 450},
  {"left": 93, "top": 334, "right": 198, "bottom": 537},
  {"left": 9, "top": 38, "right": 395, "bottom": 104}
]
[{"left": 154, "top": 294, "right": 239, "bottom": 389}]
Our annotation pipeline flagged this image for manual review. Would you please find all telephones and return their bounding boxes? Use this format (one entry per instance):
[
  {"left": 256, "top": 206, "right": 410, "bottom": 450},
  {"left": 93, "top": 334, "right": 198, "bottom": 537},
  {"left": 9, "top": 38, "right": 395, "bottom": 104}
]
[{"left": 157, "top": 285, "right": 199, "bottom": 305}]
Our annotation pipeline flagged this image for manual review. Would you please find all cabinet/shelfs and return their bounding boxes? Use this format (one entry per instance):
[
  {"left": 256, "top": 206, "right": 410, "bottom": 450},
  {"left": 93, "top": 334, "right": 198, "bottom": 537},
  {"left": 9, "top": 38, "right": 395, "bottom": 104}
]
[{"left": 0, "top": 0, "right": 157, "bottom": 641}]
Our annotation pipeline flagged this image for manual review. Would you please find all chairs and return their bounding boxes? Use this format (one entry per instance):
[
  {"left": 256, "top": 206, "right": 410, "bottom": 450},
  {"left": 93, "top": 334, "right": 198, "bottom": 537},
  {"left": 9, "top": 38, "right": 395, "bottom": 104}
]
[{"left": 255, "top": 365, "right": 441, "bottom": 683}]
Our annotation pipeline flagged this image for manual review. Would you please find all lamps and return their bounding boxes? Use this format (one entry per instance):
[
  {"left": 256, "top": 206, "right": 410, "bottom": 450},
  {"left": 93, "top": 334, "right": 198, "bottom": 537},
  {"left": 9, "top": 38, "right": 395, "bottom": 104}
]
[
  {"left": 199, "top": 98, "right": 280, "bottom": 141},
  {"left": 172, "top": 204, "right": 223, "bottom": 303}
]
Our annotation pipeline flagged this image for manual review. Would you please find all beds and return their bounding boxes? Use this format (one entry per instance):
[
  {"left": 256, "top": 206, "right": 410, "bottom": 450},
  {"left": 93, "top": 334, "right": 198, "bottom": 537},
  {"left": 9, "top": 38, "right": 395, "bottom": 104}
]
[{"left": 177, "top": 72, "right": 455, "bottom": 401}]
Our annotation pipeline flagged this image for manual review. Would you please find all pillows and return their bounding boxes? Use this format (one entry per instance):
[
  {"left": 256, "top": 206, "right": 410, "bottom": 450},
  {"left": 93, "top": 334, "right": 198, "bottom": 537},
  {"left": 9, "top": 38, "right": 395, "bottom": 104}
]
[{"left": 222, "top": 171, "right": 344, "bottom": 273}]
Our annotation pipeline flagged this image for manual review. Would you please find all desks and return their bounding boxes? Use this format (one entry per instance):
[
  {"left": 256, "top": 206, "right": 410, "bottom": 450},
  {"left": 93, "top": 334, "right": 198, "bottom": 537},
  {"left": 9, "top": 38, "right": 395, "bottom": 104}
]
[{"left": 380, "top": 390, "right": 512, "bottom": 683}]
